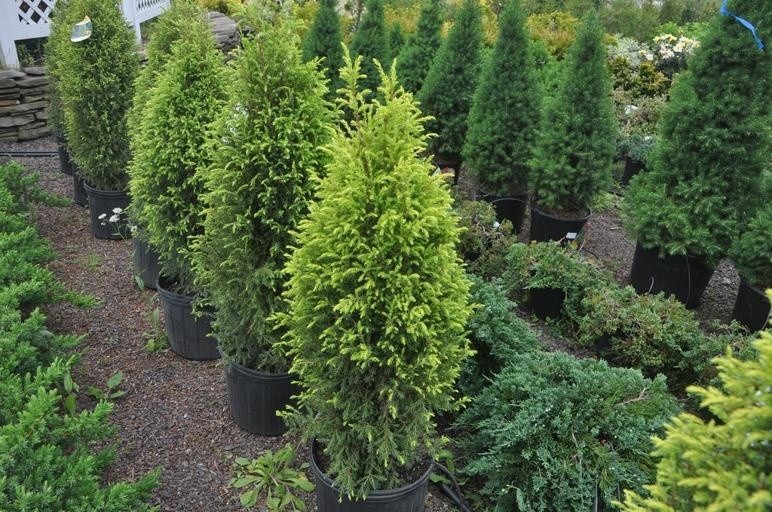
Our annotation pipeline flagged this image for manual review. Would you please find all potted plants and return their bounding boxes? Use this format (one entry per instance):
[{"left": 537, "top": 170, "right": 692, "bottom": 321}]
[
  {"left": 178, "top": 30, "right": 347, "bottom": 439},
  {"left": 237, "top": 2, "right": 770, "bottom": 511},
  {"left": 42, "top": 0, "right": 143, "bottom": 240},
  {"left": 273, "top": 86, "right": 476, "bottom": 510},
  {"left": 126, "top": 0, "right": 234, "bottom": 361}
]
